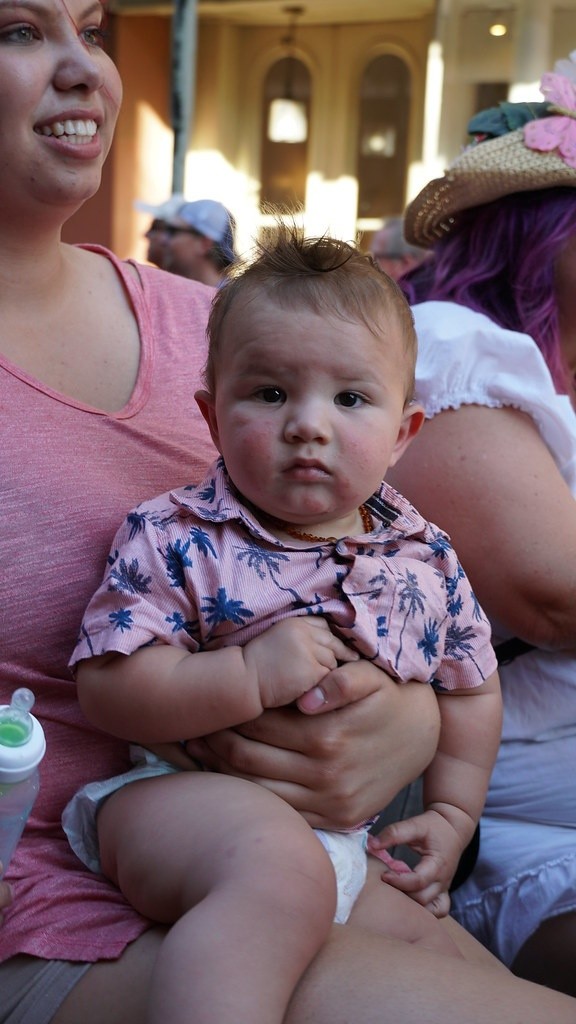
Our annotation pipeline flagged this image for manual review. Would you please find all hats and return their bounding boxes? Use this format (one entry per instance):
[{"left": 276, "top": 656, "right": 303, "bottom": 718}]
[
  {"left": 403, "top": 71, "right": 576, "bottom": 248},
  {"left": 175, "top": 199, "right": 238, "bottom": 261},
  {"left": 134, "top": 197, "right": 185, "bottom": 228}
]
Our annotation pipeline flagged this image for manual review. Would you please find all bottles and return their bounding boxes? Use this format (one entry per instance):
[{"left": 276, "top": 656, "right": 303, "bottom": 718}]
[{"left": 0, "top": 687, "right": 46, "bottom": 882}]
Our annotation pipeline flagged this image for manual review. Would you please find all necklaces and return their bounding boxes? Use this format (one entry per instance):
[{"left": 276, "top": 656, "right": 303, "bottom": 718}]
[{"left": 253, "top": 503, "right": 374, "bottom": 543}]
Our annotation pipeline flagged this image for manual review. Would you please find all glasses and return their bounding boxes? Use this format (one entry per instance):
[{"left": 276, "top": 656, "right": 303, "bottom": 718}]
[{"left": 165, "top": 226, "right": 178, "bottom": 236}]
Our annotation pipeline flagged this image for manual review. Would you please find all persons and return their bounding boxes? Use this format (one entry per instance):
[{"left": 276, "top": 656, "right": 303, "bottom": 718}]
[
  {"left": 0, "top": 0, "right": 576, "bottom": 1024},
  {"left": 136, "top": 199, "right": 238, "bottom": 286},
  {"left": 387, "top": 190, "right": 576, "bottom": 995},
  {"left": 60, "top": 241, "right": 502, "bottom": 1024},
  {"left": 367, "top": 220, "right": 432, "bottom": 276}
]
